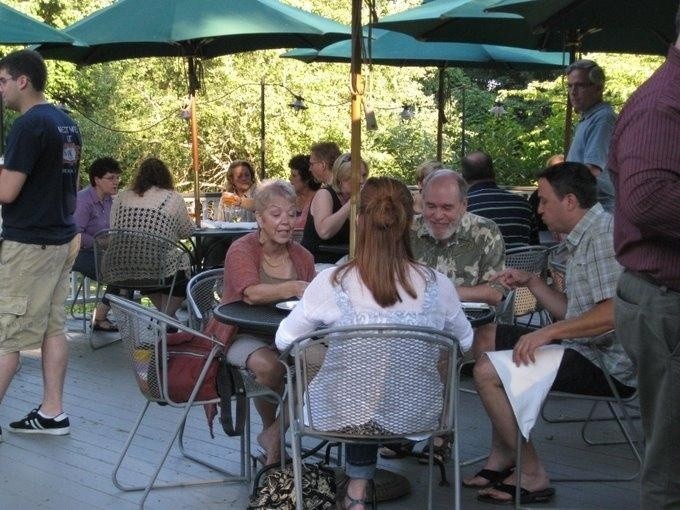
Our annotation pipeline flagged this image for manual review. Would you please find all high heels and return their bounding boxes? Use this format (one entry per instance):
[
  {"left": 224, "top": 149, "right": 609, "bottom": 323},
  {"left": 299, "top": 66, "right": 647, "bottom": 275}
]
[{"left": 250, "top": 445, "right": 269, "bottom": 468}]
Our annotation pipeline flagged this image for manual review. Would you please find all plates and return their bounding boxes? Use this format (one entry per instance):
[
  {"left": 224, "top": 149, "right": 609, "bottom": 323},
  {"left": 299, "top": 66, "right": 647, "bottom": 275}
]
[
  {"left": 461, "top": 303, "right": 488, "bottom": 311},
  {"left": 276, "top": 300, "right": 299, "bottom": 311}
]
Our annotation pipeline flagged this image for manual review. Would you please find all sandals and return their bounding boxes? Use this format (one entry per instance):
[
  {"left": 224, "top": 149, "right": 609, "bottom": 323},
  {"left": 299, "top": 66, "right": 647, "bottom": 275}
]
[
  {"left": 340, "top": 491, "right": 368, "bottom": 510},
  {"left": 89, "top": 318, "right": 119, "bottom": 332},
  {"left": 418, "top": 432, "right": 454, "bottom": 465},
  {"left": 380, "top": 443, "right": 417, "bottom": 459}
]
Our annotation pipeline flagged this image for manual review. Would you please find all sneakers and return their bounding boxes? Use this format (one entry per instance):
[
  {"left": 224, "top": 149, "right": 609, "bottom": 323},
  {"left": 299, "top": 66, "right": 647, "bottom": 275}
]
[
  {"left": 7, "top": 404, "right": 70, "bottom": 435},
  {"left": 1, "top": 427, "right": 3, "bottom": 443}
]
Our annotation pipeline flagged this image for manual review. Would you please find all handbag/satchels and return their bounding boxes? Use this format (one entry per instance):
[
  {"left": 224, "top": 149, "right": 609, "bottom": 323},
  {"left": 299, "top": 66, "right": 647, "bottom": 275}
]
[
  {"left": 147, "top": 332, "right": 247, "bottom": 437},
  {"left": 246, "top": 462, "right": 337, "bottom": 510}
]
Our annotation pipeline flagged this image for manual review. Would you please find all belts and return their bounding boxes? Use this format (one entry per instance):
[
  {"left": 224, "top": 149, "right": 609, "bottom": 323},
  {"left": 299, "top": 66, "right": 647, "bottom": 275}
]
[{"left": 625, "top": 268, "right": 680, "bottom": 294}]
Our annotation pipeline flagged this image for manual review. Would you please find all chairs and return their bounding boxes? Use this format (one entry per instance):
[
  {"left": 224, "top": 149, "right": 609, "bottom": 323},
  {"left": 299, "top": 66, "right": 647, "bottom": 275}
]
[
  {"left": 104, "top": 293, "right": 285, "bottom": 510},
  {"left": 504, "top": 244, "right": 553, "bottom": 327},
  {"left": 89, "top": 228, "right": 194, "bottom": 349},
  {"left": 185, "top": 269, "right": 224, "bottom": 326},
  {"left": 277, "top": 320, "right": 476, "bottom": 510},
  {"left": 516, "top": 330, "right": 646, "bottom": 483},
  {"left": 67, "top": 257, "right": 93, "bottom": 334},
  {"left": 548, "top": 257, "right": 568, "bottom": 294}
]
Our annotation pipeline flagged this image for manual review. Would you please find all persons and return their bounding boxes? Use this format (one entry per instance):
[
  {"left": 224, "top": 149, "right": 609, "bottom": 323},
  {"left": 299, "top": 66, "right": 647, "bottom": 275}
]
[
  {"left": 274, "top": 178, "right": 475, "bottom": 510},
  {"left": 405, "top": 170, "right": 505, "bottom": 465},
  {"left": 563, "top": 60, "right": 616, "bottom": 211},
  {"left": 411, "top": 161, "right": 444, "bottom": 212},
  {"left": 529, "top": 155, "right": 564, "bottom": 232},
  {"left": 462, "top": 162, "right": 638, "bottom": 503},
  {"left": 288, "top": 142, "right": 368, "bottom": 274},
  {"left": 0, "top": 49, "right": 81, "bottom": 434},
  {"left": 463, "top": 152, "right": 540, "bottom": 250},
  {"left": 202, "top": 180, "right": 314, "bottom": 467},
  {"left": 75, "top": 157, "right": 134, "bottom": 333},
  {"left": 609, "top": 11, "right": 680, "bottom": 510},
  {"left": 192, "top": 161, "right": 261, "bottom": 274},
  {"left": 98, "top": 157, "right": 196, "bottom": 334}
]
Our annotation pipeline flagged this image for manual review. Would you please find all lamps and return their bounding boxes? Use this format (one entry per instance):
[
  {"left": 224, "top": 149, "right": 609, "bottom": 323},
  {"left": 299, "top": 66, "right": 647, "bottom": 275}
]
[
  {"left": 488, "top": 103, "right": 508, "bottom": 117},
  {"left": 56, "top": 104, "right": 70, "bottom": 114},
  {"left": 399, "top": 108, "right": 415, "bottom": 121},
  {"left": 288, "top": 97, "right": 308, "bottom": 112},
  {"left": 180, "top": 103, "right": 191, "bottom": 124}
]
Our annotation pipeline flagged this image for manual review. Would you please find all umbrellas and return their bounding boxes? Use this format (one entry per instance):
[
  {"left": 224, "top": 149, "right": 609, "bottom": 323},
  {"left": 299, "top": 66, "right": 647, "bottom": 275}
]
[
  {"left": 24, "top": 0, "right": 377, "bottom": 224},
  {"left": 279, "top": 0, "right": 570, "bottom": 160},
  {"left": 0, "top": 0, "right": 89, "bottom": 45},
  {"left": 368, "top": 1, "right": 676, "bottom": 161},
  {"left": 482, "top": 2, "right": 680, "bottom": 29}
]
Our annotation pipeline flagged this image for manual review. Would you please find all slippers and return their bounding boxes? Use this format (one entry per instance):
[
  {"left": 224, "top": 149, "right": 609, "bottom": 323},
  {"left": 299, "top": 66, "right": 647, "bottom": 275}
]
[
  {"left": 477, "top": 481, "right": 557, "bottom": 505},
  {"left": 462, "top": 469, "right": 515, "bottom": 488}
]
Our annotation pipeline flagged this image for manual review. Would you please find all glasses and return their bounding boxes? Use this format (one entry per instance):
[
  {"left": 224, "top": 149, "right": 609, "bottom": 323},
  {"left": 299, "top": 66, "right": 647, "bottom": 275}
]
[
  {"left": 97, "top": 175, "right": 122, "bottom": 182},
  {"left": 308, "top": 160, "right": 329, "bottom": 166},
  {"left": 0, "top": 73, "right": 32, "bottom": 85}
]
